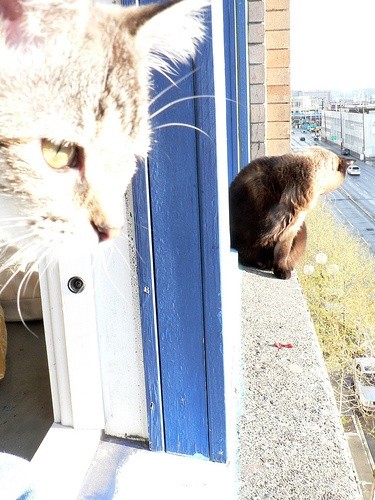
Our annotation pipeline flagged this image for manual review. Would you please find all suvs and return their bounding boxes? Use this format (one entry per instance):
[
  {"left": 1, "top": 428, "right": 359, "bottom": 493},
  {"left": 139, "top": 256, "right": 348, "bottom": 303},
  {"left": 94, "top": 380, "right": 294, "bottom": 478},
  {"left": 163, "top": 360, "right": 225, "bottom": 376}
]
[{"left": 341, "top": 149, "right": 350, "bottom": 155}]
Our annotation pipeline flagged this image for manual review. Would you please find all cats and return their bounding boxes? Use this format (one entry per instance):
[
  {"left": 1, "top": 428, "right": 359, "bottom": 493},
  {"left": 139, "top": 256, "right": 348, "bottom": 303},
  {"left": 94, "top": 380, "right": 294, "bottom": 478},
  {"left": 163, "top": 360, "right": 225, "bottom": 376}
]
[
  {"left": 0, "top": 1, "right": 216, "bottom": 343},
  {"left": 228, "top": 146, "right": 357, "bottom": 280}
]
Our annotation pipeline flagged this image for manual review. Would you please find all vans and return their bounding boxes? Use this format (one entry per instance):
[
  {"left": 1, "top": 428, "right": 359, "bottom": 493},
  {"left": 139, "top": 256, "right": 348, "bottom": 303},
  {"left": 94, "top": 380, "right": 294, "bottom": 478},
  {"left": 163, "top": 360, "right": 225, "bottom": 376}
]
[{"left": 346, "top": 165, "right": 361, "bottom": 175}]
